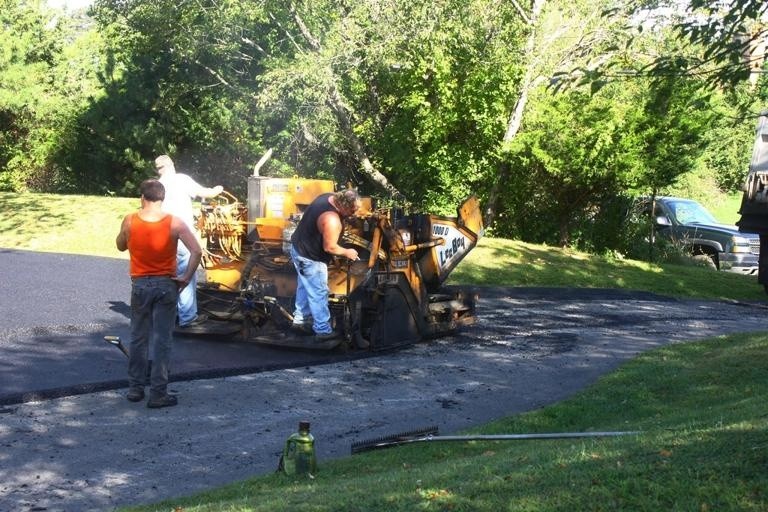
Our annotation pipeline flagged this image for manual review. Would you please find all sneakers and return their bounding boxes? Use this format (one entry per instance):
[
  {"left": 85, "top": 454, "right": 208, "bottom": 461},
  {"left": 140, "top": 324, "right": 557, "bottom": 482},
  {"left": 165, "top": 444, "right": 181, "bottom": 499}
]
[
  {"left": 182, "top": 313, "right": 208, "bottom": 327},
  {"left": 312, "top": 330, "right": 340, "bottom": 343},
  {"left": 127, "top": 390, "right": 145, "bottom": 401},
  {"left": 147, "top": 394, "right": 177, "bottom": 407},
  {"left": 289, "top": 323, "right": 315, "bottom": 335}
]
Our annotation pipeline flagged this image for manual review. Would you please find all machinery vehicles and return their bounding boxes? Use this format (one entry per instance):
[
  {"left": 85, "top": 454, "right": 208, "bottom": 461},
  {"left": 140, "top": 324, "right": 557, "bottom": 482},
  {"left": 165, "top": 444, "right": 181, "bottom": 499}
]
[{"left": 172, "top": 146, "right": 487, "bottom": 355}]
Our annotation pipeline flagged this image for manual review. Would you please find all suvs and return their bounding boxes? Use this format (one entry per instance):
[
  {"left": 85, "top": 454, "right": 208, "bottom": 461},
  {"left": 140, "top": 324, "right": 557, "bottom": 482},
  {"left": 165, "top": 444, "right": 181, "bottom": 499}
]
[{"left": 630, "top": 193, "right": 761, "bottom": 272}]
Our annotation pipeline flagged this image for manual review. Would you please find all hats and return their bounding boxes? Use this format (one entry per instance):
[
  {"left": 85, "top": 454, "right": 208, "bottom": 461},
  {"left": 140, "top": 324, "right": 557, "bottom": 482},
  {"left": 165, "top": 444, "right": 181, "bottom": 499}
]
[{"left": 153, "top": 155, "right": 170, "bottom": 169}]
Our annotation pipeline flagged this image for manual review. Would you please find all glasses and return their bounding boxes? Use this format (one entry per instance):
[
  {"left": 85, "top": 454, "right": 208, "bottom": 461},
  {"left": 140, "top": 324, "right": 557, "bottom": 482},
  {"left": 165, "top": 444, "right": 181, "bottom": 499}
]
[{"left": 299, "top": 261, "right": 304, "bottom": 275}]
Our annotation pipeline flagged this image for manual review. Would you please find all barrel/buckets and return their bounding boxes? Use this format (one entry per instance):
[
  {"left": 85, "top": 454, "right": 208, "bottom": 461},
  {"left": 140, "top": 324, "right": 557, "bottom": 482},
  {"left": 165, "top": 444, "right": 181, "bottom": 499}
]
[{"left": 281, "top": 421, "right": 317, "bottom": 476}]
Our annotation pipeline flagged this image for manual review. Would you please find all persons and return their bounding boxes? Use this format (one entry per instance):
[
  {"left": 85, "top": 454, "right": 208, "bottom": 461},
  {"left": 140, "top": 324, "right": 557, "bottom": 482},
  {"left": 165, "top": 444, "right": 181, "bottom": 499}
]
[
  {"left": 155, "top": 155, "right": 224, "bottom": 328},
  {"left": 290, "top": 189, "right": 360, "bottom": 342},
  {"left": 116, "top": 179, "right": 203, "bottom": 409}
]
